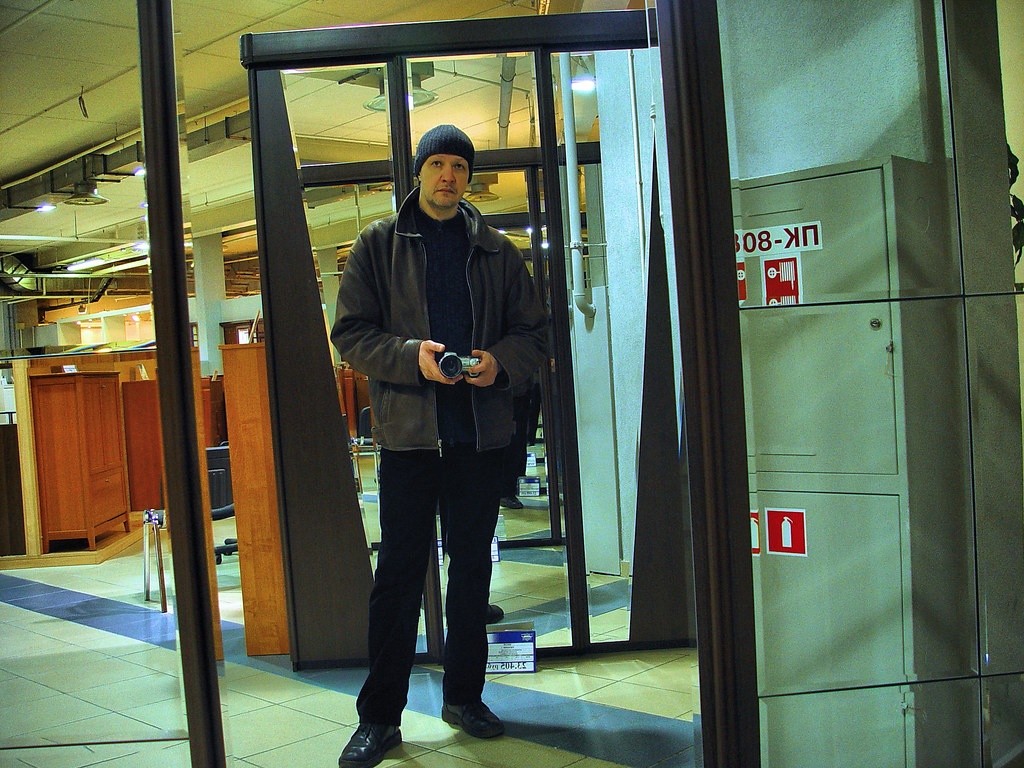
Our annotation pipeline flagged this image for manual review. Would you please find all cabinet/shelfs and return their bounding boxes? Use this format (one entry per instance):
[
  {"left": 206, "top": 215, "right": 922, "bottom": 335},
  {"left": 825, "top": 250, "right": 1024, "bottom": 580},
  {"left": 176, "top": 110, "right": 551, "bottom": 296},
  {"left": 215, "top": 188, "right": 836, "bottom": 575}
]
[
  {"left": 29, "top": 372, "right": 130, "bottom": 553},
  {"left": 731, "top": 155, "right": 977, "bottom": 768},
  {"left": 120, "top": 378, "right": 227, "bottom": 511}
]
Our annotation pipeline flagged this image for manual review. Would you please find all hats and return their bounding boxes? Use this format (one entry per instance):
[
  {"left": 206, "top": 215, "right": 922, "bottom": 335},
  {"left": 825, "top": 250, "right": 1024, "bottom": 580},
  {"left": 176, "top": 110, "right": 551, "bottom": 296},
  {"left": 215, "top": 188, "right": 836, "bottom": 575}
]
[{"left": 413, "top": 124, "right": 474, "bottom": 184}]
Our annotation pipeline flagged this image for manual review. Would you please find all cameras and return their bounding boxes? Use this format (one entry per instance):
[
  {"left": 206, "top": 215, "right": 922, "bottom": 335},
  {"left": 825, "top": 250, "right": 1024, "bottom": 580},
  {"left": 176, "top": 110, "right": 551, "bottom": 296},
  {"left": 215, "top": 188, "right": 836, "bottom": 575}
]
[{"left": 438, "top": 351, "right": 482, "bottom": 378}]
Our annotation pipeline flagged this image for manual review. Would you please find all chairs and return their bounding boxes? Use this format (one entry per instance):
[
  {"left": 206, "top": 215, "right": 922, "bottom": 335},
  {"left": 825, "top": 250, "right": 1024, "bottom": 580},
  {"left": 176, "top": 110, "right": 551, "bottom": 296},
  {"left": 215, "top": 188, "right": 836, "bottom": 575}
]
[
  {"left": 353, "top": 406, "right": 381, "bottom": 494},
  {"left": 144, "top": 446, "right": 240, "bottom": 613}
]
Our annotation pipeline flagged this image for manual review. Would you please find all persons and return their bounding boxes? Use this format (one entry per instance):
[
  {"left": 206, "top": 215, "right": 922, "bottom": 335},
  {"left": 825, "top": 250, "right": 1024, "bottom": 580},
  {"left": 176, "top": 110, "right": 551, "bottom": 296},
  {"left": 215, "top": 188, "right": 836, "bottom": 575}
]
[{"left": 330, "top": 125, "right": 548, "bottom": 768}]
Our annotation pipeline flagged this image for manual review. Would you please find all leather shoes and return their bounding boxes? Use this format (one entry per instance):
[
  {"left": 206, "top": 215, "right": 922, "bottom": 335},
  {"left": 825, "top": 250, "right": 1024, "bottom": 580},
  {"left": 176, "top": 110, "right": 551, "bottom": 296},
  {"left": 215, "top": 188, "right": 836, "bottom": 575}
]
[
  {"left": 486, "top": 603, "right": 504, "bottom": 624},
  {"left": 442, "top": 697, "right": 505, "bottom": 739},
  {"left": 338, "top": 722, "right": 402, "bottom": 768},
  {"left": 499, "top": 495, "right": 523, "bottom": 509}
]
